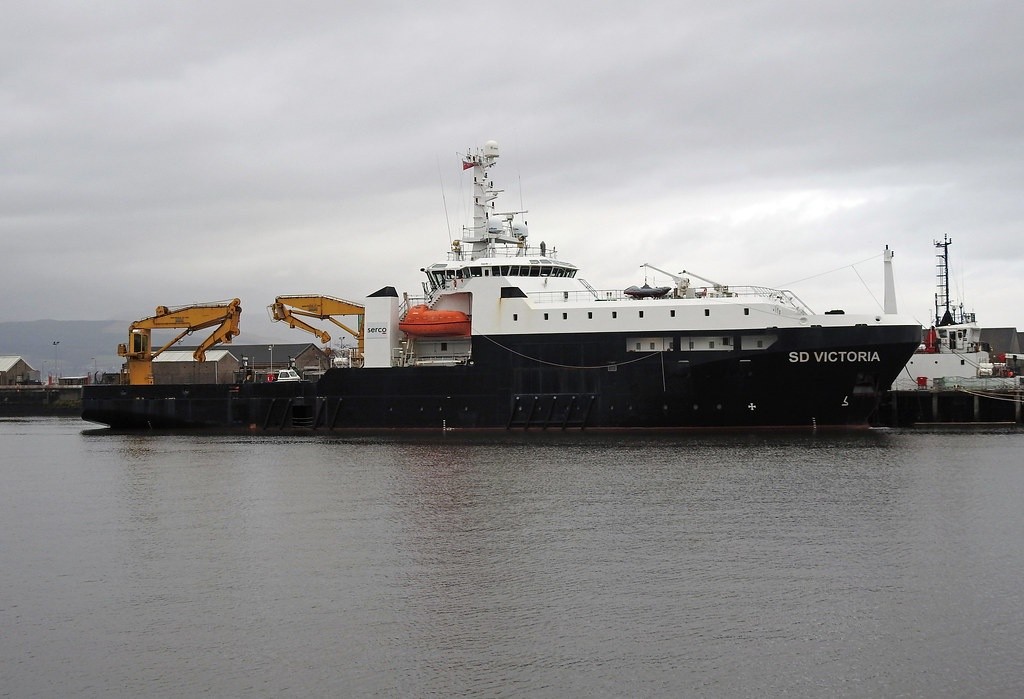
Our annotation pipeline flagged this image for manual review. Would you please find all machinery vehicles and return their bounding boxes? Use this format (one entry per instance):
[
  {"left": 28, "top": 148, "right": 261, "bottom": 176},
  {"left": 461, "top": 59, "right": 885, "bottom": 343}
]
[
  {"left": 118, "top": 299, "right": 241, "bottom": 385},
  {"left": 267, "top": 294, "right": 364, "bottom": 359}
]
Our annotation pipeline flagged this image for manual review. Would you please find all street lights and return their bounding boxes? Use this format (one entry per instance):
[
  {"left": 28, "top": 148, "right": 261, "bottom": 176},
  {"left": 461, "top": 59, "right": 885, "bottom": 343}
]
[
  {"left": 91, "top": 358, "right": 96, "bottom": 371},
  {"left": 42, "top": 361, "right": 47, "bottom": 381}
]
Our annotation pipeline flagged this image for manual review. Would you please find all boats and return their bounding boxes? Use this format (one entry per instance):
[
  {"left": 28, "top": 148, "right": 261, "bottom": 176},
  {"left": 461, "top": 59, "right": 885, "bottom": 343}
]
[
  {"left": 877, "top": 233, "right": 1024, "bottom": 427},
  {"left": 80, "top": 141, "right": 922, "bottom": 435}
]
